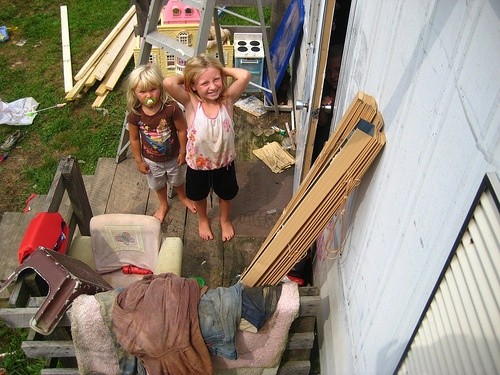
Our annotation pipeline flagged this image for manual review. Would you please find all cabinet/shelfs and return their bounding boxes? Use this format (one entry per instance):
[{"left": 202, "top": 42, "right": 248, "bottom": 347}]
[{"left": 233, "top": 33, "right": 264, "bottom": 93}]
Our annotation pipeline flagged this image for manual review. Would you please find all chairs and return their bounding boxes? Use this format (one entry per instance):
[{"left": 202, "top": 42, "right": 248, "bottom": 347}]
[{"left": 90, "top": 213, "right": 161, "bottom": 288}]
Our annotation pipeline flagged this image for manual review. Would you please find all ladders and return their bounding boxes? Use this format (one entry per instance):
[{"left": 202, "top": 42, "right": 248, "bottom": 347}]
[{"left": 114, "top": 0, "right": 281, "bottom": 199}]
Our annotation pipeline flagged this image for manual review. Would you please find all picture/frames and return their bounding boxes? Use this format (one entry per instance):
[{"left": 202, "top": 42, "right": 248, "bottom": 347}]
[{"left": 264, "top": 0, "right": 305, "bottom": 102}]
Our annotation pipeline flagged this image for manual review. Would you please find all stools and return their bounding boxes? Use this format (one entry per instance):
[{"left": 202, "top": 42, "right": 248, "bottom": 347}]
[{"left": 0, "top": 247, "right": 115, "bottom": 335}]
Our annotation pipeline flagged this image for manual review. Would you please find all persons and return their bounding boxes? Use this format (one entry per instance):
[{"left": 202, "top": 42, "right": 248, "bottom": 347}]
[
  {"left": 162, "top": 56, "right": 251, "bottom": 242},
  {"left": 127, "top": 63, "right": 197, "bottom": 222}
]
